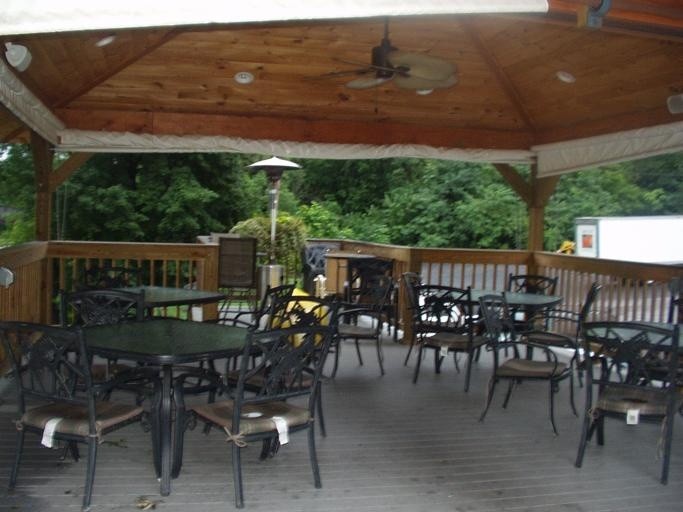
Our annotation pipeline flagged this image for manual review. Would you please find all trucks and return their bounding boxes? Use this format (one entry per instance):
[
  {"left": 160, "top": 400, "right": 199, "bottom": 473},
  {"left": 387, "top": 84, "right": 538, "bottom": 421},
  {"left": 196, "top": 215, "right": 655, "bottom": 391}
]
[{"left": 575, "top": 213, "right": 683, "bottom": 266}]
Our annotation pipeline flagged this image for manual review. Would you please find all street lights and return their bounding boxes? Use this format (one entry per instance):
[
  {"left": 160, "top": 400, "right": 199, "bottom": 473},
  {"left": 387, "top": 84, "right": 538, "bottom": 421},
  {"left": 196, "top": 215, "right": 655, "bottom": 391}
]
[{"left": 245, "top": 155, "right": 301, "bottom": 309}]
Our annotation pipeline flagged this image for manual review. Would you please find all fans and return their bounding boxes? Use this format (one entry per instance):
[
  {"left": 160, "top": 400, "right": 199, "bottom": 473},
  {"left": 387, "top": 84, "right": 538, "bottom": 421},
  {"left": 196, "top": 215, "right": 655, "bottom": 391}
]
[{"left": 315, "top": 15, "right": 462, "bottom": 90}]
